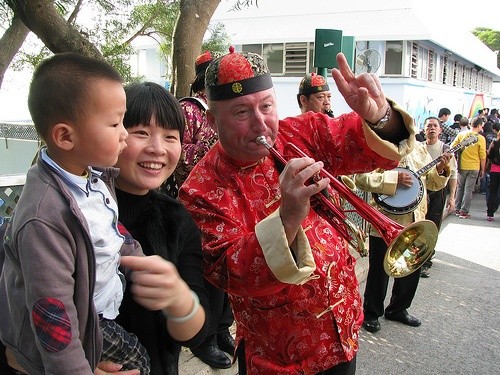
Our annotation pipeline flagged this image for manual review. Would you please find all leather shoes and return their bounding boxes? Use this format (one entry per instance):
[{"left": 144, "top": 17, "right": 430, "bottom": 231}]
[
  {"left": 385, "top": 310, "right": 421, "bottom": 327},
  {"left": 190, "top": 335, "right": 231, "bottom": 369},
  {"left": 218, "top": 331, "right": 239, "bottom": 354},
  {"left": 362, "top": 319, "right": 381, "bottom": 333}
]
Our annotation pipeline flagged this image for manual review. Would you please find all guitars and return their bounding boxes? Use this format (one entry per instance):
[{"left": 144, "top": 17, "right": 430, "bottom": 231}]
[{"left": 373, "top": 133, "right": 478, "bottom": 215}]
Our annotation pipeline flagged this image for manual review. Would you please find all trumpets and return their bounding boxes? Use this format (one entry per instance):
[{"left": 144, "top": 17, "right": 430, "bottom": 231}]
[{"left": 258, "top": 135, "right": 439, "bottom": 279}]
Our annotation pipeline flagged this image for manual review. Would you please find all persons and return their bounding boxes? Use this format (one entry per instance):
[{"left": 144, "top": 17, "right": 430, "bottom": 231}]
[
  {"left": 356, "top": 141, "right": 450, "bottom": 332},
  {"left": 419, "top": 117, "right": 458, "bottom": 277},
  {"left": 0, "top": 53, "right": 152, "bottom": 375},
  {"left": 178, "top": 46, "right": 417, "bottom": 375},
  {"left": 438, "top": 108, "right": 500, "bottom": 220},
  {"left": 0, "top": 82, "right": 210, "bottom": 375},
  {"left": 450, "top": 117, "right": 486, "bottom": 218},
  {"left": 174, "top": 50, "right": 235, "bottom": 369},
  {"left": 297, "top": 72, "right": 332, "bottom": 114}
]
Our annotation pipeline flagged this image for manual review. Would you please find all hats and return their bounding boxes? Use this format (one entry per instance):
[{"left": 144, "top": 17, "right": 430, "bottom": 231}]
[
  {"left": 194, "top": 49, "right": 225, "bottom": 72},
  {"left": 205, "top": 45, "right": 274, "bottom": 101},
  {"left": 299, "top": 72, "right": 329, "bottom": 95}
]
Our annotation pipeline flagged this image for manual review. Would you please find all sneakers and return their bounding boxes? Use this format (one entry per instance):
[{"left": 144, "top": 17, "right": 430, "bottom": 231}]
[{"left": 420, "top": 264, "right": 431, "bottom": 277}]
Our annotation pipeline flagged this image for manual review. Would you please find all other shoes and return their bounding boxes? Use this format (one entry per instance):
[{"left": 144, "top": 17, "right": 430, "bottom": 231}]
[
  {"left": 486, "top": 215, "right": 494, "bottom": 221},
  {"left": 458, "top": 210, "right": 471, "bottom": 218},
  {"left": 455, "top": 210, "right": 461, "bottom": 216}
]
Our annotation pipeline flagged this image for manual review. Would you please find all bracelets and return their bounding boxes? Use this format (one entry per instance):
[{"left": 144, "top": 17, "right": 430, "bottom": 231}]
[{"left": 167, "top": 290, "right": 200, "bottom": 322}]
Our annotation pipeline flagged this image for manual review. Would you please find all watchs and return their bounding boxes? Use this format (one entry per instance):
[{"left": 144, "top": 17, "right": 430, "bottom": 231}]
[{"left": 365, "top": 108, "right": 391, "bottom": 129}]
